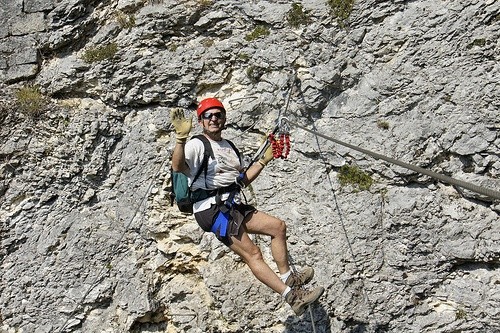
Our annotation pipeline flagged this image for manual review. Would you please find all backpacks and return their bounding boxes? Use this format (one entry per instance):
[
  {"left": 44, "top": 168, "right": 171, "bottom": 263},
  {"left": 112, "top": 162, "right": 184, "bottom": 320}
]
[{"left": 166, "top": 134, "right": 241, "bottom": 212}]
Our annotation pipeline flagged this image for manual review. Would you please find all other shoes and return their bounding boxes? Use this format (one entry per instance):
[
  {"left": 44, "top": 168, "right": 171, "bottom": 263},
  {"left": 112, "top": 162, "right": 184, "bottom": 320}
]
[
  {"left": 286, "top": 286, "right": 326, "bottom": 316},
  {"left": 284, "top": 266, "right": 314, "bottom": 290}
]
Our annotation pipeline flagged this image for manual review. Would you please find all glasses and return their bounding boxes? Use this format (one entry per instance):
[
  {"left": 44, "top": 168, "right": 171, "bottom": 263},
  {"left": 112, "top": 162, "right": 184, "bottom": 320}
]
[{"left": 200, "top": 110, "right": 224, "bottom": 119}]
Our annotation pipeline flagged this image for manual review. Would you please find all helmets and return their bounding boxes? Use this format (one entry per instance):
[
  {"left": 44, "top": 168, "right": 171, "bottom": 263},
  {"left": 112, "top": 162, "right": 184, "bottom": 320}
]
[{"left": 196, "top": 97, "right": 226, "bottom": 118}]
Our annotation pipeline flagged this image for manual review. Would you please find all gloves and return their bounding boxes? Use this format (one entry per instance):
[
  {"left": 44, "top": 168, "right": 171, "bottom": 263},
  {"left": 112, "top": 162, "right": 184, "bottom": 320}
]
[
  {"left": 257, "top": 133, "right": 279, "bottom": 166},
  {"left": 170, "top": 107, "right": 192, "bottom": 143}
]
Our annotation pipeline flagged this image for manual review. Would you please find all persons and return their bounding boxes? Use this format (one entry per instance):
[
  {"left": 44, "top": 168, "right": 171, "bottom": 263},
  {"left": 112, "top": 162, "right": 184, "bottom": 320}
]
[{"left": 171, "top": 98, "right": 325, "bottom": 316}]
